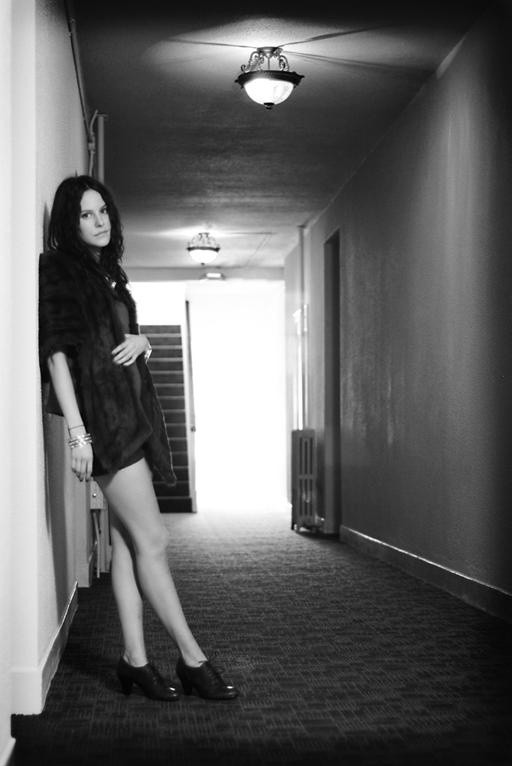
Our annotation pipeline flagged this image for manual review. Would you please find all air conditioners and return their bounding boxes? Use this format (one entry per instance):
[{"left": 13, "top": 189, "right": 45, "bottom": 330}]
[{"left": 291, "top": 428, "right": 316, "bottom": 533}]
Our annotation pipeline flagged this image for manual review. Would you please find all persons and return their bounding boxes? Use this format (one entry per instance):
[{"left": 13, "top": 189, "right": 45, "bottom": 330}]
[{"left": 39, "top": 177, "right": 238, "bottom": 701}]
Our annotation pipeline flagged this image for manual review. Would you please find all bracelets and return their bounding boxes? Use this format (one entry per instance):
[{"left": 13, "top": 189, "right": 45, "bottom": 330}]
[
  {"left": 69, "top": 424, "right": 84, "bottom": 431},
  {"left": 68, "top": 431, "right": 93, "bottom": 450}
]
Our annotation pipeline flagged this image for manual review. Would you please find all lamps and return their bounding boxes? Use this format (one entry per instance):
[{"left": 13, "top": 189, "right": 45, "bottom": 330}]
[
  {"left": 186, "top": 232, "right": 221, "bottom": 267},
  {"left": 234, "top": 47, "right": 305, "bottom": 109}
]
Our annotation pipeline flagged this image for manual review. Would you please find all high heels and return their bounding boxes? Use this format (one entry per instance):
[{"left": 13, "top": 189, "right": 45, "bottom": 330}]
[
  {"left": 175, "top": 654, "right": 239, "bottom": 701},
  {"left": 116, "top": 656, "right": 179, "bottom": 701}
]
